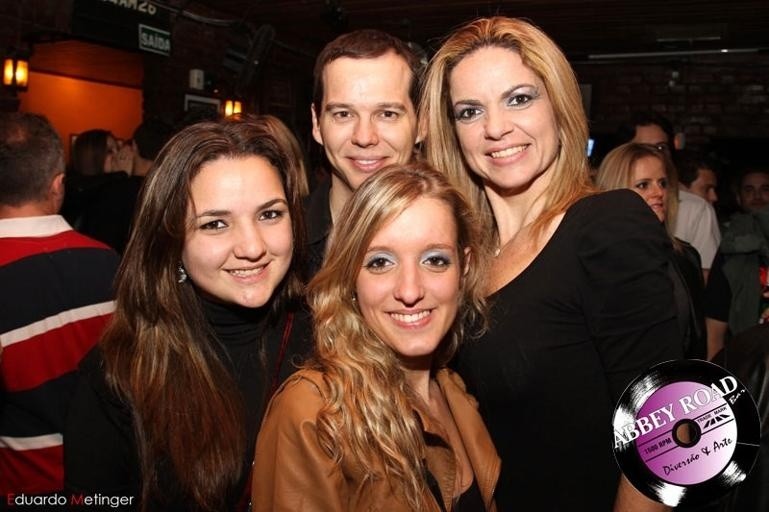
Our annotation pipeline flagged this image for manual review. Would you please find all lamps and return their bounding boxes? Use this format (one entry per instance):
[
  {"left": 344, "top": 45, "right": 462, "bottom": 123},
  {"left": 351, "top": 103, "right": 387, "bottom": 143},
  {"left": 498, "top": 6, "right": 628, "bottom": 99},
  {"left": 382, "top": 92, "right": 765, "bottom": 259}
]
[{"left": 0, "top": 50, "right": 30, "bottom": 113}]
